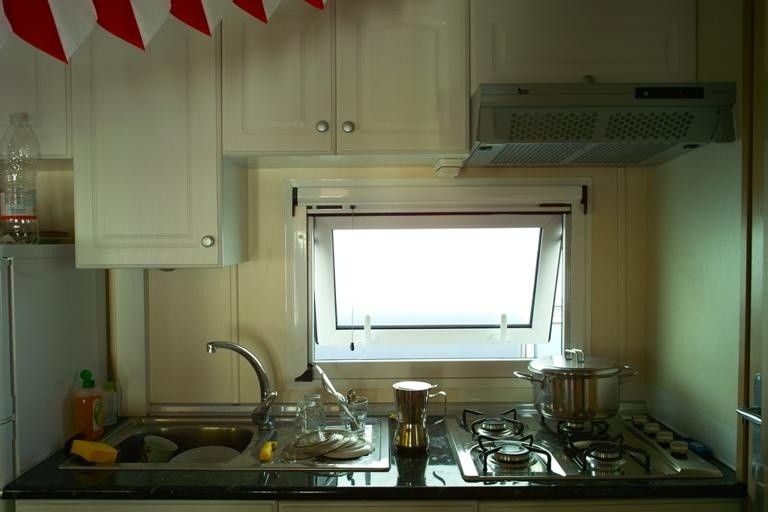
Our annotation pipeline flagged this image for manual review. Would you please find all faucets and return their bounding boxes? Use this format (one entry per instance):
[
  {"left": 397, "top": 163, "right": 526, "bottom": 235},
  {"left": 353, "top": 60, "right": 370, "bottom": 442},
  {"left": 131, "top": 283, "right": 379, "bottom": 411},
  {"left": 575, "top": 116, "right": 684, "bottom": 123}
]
[{"left": 206, "top": 340, "right": 277, "bottom": 424}]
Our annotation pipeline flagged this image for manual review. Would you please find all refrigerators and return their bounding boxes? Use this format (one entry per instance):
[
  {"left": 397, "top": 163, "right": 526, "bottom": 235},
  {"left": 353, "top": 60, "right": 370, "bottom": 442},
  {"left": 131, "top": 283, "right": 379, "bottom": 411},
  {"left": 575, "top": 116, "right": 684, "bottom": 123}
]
[{"left": 0, "top": 244, "right": 108, "bottom": 512}]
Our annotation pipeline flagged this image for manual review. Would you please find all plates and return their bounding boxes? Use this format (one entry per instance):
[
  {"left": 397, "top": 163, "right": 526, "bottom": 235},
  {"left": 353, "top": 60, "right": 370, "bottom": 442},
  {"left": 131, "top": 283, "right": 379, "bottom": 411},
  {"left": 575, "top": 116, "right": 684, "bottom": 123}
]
[
  {"left": 280, "top": 427, "right": 375, "bottom": 459},
  {"left": 28, "top": 230, "right": 72, "bottom": 243}
]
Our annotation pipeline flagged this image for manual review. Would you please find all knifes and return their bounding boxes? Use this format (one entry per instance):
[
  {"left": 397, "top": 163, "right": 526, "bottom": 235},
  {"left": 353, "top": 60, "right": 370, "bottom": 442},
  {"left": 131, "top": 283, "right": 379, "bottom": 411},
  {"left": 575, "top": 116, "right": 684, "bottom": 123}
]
[{"left": 259, "top": 430, "right": 278, "bottom": 461}]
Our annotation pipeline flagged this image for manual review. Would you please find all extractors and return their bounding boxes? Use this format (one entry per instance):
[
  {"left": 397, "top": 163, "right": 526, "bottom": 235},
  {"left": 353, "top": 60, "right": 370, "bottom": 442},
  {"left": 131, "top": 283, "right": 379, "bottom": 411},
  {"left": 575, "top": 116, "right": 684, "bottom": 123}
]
[{"left": 466, "top": 81, "right": 737, "bottom": 169}]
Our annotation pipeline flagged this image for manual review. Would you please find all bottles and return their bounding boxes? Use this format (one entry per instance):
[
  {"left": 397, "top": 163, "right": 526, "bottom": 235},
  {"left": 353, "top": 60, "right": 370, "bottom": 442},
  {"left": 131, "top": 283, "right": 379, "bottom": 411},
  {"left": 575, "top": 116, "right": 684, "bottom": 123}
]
[
  {"left": 1, "top": 112, "right": 40, "bottom": 244},
  {"left": 73, "top": 367, "right": 119, "bottom": 443}
]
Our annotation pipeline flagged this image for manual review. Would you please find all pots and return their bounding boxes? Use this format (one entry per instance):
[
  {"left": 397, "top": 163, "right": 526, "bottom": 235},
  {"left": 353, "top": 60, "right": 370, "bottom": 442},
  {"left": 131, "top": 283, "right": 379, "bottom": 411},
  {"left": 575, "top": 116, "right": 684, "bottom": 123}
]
[{"left": 513, "top": 347, "right": 641, "bottom": 421}]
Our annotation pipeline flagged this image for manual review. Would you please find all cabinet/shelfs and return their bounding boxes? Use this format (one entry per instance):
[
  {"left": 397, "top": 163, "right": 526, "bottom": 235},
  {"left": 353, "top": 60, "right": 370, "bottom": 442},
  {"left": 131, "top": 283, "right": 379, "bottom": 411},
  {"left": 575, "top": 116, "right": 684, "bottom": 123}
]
[
  {"left": 222, "top": 0, "right": 470, "bottom": 168},
  {"left": 74, "top": 1, "right": 249, "bottom": 270}
]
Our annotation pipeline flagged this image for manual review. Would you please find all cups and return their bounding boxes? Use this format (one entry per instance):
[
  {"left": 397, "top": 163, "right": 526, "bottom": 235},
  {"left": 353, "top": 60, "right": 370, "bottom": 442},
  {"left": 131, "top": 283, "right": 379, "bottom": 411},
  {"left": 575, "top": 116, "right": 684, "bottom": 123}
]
[
  {"left": 338, "top": 395, "right": 370, "bottom": 437},
  {"left": 292, "top": 392, "right": 328, "bottom": 439},
  {"left": 137, "top": 434, "right": 179, "bottom": 463}
]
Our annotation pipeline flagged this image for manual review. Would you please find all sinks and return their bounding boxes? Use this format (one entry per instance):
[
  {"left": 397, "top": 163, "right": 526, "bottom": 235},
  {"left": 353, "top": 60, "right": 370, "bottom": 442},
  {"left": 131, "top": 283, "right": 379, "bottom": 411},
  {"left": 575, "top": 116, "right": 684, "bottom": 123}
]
[{"left": 60, "top": 417, "right": 253, "bottom": 472}]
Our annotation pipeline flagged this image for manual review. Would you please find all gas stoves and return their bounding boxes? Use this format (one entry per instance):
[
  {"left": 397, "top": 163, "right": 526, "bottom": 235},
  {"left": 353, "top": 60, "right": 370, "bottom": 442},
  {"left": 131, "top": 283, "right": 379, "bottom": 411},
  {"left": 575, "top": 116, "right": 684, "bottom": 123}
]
[{"left": 444, "top": 412, "right": 725, "bottom": 481}]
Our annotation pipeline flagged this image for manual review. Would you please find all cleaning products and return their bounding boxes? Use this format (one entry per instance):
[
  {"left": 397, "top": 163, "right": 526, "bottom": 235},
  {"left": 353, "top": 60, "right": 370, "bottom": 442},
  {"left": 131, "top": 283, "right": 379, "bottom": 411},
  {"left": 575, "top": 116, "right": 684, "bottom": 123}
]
[{"left": 75, "top": 370, "right": 103, "bottom": 440}]
